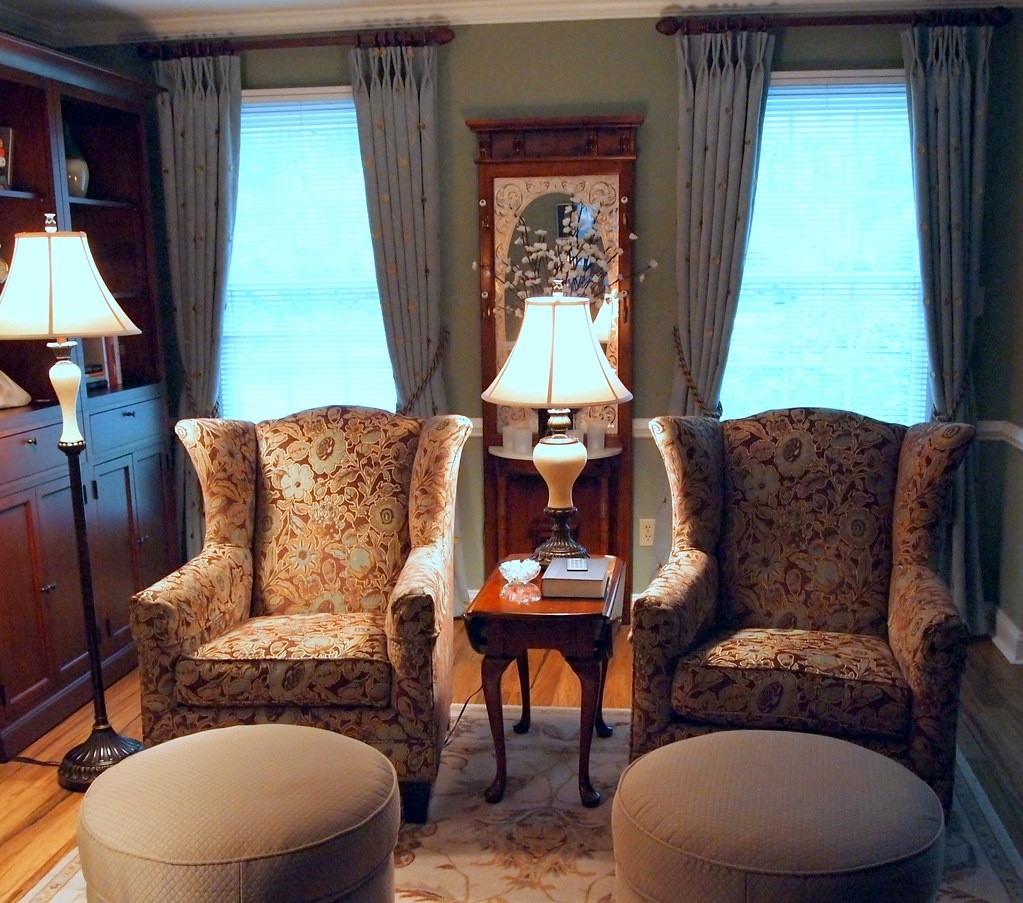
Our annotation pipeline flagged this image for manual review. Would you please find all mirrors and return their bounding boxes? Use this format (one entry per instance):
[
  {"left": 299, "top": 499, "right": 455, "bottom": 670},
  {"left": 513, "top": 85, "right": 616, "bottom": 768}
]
[{"left": 504, "top": 193, "right": 612, "bottom": 356}]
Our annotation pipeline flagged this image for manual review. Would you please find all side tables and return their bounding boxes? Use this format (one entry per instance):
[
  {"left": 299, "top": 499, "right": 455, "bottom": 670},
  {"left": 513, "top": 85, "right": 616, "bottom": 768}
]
[{"left": 461, "top": 553, "right": 626, "bottom": 809}]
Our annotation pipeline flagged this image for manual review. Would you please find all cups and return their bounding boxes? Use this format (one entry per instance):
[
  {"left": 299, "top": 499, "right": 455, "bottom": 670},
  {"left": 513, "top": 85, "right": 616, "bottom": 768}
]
[
  {"left": 502, "top": 424, "right": 517, "bottom": 450},
  {"left": 513, "top": 428, "right": 532, "bottom": 455},
  {"left": 586, "top": 420, "right": 606, "bottom": 452},
  {"left": 566, "top": 429, "right": 584, "bottom": 446}
]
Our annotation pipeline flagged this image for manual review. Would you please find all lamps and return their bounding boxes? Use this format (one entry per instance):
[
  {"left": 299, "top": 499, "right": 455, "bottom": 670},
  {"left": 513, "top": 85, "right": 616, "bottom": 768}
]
[
  {"left": 480, "top": 279, "right": 635, "bottom": 570},
  {"left": 0, "top": 212, "right": 142, "bottom": 793}
]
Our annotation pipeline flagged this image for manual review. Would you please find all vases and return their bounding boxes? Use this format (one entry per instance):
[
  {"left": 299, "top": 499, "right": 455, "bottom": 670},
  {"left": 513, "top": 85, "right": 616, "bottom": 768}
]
[{"left": 533, "top": 408, "right": 581, "bottom": 441}]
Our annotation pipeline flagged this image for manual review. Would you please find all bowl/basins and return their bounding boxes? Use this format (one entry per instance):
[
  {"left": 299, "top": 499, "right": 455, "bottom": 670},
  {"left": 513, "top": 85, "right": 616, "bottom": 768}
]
[
  {"left": 496, "top": 582, "right": 542, "bottom": 602},
  {"left": 495, "top": 557, "right": 542, "bottom": 583}
]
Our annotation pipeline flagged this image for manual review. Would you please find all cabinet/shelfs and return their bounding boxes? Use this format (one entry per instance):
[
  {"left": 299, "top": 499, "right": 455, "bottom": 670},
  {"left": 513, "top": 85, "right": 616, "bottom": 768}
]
[{"left": 0, "top": 32, "right": 189, "bottom": 765}]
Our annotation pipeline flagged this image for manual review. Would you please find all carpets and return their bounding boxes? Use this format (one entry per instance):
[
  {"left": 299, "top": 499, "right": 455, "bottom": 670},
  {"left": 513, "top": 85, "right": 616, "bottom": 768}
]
[{"left": 4, "top": 703, "right": 1022, "bottom": 903}]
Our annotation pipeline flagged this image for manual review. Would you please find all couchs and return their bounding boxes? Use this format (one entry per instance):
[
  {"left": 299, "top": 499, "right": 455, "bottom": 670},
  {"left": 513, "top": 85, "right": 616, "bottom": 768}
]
[
  {"left": 127, "top": 405, "right": 472, "bottom": 827},
  {"left": 630, "top": 407, "right": 978, "bottom": 806}
]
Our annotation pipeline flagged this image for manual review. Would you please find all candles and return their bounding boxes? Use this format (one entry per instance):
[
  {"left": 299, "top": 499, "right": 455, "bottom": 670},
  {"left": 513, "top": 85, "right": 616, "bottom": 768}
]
[
  {"left": 514, "top": 428, "right": 532, "bottom": 455},
  {"left": 587, "top": 425, "right": 605, "bottom": 452},
  {"left": 502, "top": 424, "right": 513, "bottom": 448}
]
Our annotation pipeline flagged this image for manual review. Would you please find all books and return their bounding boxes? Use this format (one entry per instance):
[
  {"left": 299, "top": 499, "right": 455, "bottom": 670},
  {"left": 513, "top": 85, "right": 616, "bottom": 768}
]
[
  {"left": 82, "top": 336, "right": 123, "bottom": 392},
  {"left": 539, "top": 557, "right": 610, "bottom": 600}
]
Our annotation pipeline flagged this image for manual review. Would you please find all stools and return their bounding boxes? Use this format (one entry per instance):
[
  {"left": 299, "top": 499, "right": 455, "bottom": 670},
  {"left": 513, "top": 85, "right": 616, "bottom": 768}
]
[
  {"left": 608, "top": 729, "right": 946, "bottom": 903},
  {"left": 76, "top": 723, "right": 404, "bottom": 903}
]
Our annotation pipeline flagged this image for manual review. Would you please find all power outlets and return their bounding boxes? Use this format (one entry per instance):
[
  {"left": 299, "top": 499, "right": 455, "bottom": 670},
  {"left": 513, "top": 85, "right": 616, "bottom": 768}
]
[{"left": 639, "top": 518, "right": 655, "bottom": 547}]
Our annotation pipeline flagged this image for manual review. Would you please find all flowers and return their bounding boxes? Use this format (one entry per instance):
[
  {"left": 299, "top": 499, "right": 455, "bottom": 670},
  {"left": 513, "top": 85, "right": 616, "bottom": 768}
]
[{"left": 471, "top": 181, "right": 657, "bottom": 318}]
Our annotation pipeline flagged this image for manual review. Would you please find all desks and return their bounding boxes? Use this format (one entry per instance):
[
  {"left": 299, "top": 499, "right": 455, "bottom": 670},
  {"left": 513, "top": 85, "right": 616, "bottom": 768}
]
[{"left": 487, "top": 445, "right": 623, "bottom": 476}]
